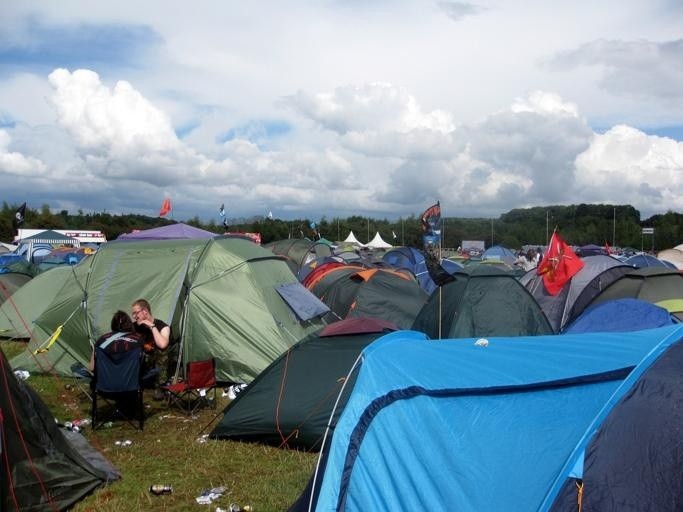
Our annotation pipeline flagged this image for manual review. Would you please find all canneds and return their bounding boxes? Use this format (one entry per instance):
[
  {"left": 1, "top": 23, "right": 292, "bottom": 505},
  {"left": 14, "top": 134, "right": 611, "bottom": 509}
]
[
  {"left": 64, "top": 421, "right": 73, "bottom": 427},
  {"left": 231, "top": 504, "right": 252, "bottom": 512},
  {"left": 73, "top": 426, "right": 83, "bottom": 432},
  {"left": 149, "top": 483, "right": 173, "bottom": 495}
]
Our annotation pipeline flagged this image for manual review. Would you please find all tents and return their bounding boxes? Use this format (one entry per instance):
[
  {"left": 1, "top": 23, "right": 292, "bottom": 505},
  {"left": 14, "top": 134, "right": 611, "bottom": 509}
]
[
  {"left": 2, "top": 218, "right": 327, "bottom": 385},
  {"left": 200, "top": 231, "right": 682, "bottom": 452},
  {"left": 1, "top": 344, "right": 122, "bottom": 511},
  {"left": 287, "top": 324, "right": 683, "bottom": 512}
]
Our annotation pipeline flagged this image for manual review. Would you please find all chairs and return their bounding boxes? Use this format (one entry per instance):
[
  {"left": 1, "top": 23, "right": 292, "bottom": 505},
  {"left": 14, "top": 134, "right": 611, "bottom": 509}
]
[
  {"left": 160, "top": 358, "right": 217, "bottom": 415},
  {"left": 91, "top": 346, "right": 144, "bottom": 429}
]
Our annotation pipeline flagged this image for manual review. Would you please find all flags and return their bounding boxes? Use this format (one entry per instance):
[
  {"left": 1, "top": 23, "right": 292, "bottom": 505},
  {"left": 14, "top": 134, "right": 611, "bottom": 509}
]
[
  {"left": 158, "top": 199, "right": 171, "bottom": 217},
  {"left": 420, "top": 204, "right": 442, "bottom": 251}
]
